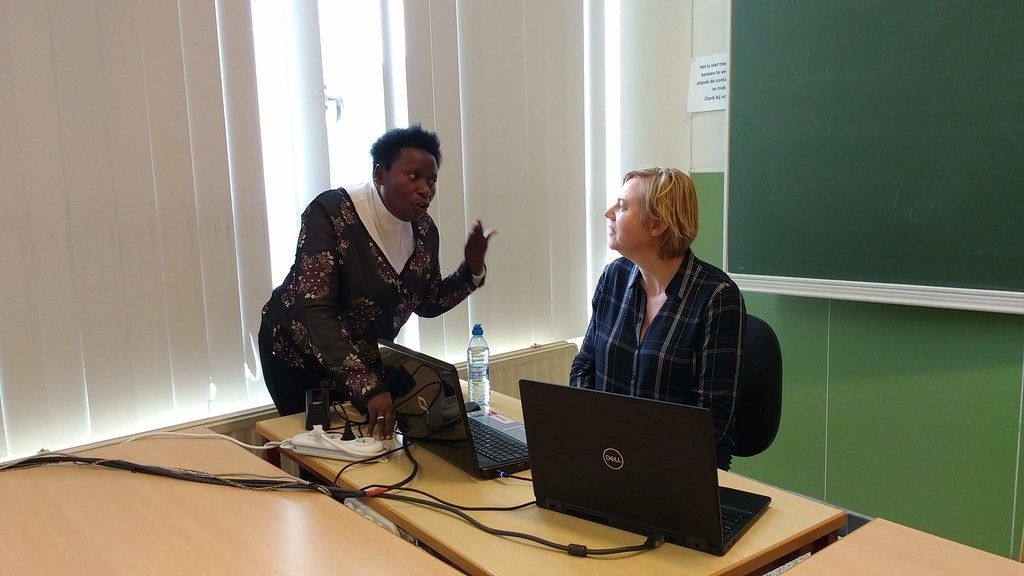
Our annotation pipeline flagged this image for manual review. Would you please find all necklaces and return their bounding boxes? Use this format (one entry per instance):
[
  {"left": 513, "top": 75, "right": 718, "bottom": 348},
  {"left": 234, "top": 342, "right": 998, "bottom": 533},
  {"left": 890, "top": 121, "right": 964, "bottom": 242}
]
[{"left": 370, "top": 185, "right": 403, "bottom": 294}]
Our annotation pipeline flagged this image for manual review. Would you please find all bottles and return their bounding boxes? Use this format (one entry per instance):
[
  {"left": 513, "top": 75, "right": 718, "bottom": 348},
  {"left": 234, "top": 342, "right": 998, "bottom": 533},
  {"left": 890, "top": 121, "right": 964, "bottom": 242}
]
[{"left": 467, "top": 324, "right": 490, "bottom": 416}]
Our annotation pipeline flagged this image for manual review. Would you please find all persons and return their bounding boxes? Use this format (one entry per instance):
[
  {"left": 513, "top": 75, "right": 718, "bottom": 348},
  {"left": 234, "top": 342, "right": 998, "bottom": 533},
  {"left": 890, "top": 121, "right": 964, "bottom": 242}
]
[
  {"left": 257, "top": 125, "right": 497, "bottom": 441},
  {"left": 570, "top": 168, "right": 746, "bottom": 470}
]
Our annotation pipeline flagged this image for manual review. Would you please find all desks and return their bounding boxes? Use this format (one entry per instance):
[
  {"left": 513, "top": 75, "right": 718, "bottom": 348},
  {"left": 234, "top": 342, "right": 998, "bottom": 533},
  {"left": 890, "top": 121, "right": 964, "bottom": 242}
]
[{"left": 0, "top": 378, "right": 1024, "bottom": 576}]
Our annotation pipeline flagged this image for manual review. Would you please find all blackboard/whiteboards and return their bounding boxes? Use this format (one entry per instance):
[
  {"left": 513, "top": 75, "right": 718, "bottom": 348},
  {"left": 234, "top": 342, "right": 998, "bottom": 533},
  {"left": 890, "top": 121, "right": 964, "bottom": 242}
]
[{"left": 721, "top": 0, "right": 1024, "bottom": 315}]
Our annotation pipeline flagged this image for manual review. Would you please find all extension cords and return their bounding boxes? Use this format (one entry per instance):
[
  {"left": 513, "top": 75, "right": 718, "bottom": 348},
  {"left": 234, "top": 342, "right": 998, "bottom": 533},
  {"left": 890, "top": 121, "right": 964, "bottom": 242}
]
[{"left": 290, "top": 433, "right": 384, "bottom": 461}]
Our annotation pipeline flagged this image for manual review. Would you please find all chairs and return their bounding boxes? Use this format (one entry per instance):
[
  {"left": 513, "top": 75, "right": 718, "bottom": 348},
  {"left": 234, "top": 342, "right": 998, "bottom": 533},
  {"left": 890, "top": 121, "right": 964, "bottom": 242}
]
[{"left": 728, "top": 313, "right": 784, "bottom": 458}]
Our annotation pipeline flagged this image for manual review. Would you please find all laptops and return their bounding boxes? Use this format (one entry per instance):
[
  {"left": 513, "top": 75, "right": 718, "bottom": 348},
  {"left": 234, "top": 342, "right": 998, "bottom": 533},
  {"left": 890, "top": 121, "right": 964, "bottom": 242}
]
[
  {"left": 519, "top": 379, "right": 772, "bottom": 557},
  {"left": 377, "top": 339, "right": 531, "bottom": 480}
]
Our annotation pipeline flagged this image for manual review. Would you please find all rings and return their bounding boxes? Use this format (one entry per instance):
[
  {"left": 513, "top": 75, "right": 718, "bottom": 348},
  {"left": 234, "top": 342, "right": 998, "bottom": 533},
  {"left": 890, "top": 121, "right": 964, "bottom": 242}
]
[{"left": 377, "top": 416, "right": 385, "bottom": 420}]
